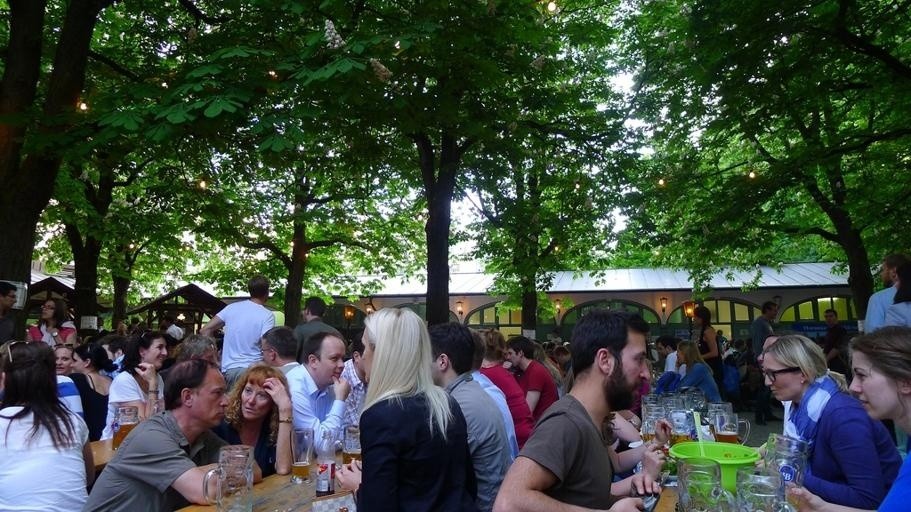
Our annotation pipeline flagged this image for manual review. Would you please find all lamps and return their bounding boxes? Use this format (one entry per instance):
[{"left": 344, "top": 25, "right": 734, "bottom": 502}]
[
  {"left": 556, "top": 299, "right": 560, "bottom": 313},
  {"left": 455, "top": 301, "right": 463, "bottom": 315},
  {"left": 365, "top": 297, "right": 372, "bottom": 315},
  {"left": 660, "top": 297, "right": 667, "bottom": 312}
]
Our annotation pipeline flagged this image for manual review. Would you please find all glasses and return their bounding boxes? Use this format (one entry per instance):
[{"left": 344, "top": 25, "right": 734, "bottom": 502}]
[
  {"left": 139, "top": 327, "right": 154, "bottom": 344},
  {"left": 260, "top": 348, "right": 275, "bottom": 354},
  {"left": 762, "top": 366, "right": 804, "bottom": 381},
  {"left": 0, "top": 293, "right": 18, "bottom": 299},
  {"left": 39, "top": 303, "right": 56, "bottom": 311},
  {"left": 54, "top": 343, "right": 73, "bottom": 347},
  {"left": 0, "top": 338, "right": 32, "bottom": 364}
]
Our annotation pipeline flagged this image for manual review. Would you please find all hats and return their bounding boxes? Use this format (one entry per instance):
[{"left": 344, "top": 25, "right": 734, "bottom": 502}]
[{"left": 654, "top": 371, "right": 681, "bottom": 394}]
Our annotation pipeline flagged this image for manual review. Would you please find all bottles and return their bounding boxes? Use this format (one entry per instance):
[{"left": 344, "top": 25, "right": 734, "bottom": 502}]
[{"left": 314, "top": 430, "right": 335, "bottom": 495}]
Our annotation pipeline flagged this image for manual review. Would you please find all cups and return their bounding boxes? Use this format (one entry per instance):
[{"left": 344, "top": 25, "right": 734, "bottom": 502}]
[
  {"left": 343, "top": 429, "right": 361, "bottom": 465},
  {"left": 287, "top": 426, "right": 316, "bottom": 483},
  {"left": 714, "top": 411, "right": 751, "bottom": 448},
  {"left": 148, "top": 399, "right": 165, "bottom": 415},
  {"left": 733, "top": 465, "right": 796, "bottom": 512},
  {"left": 706, "top": 401, "right": 734, "bottom": 436},
  {"left": 761, "top": 433, "right": 807, "bottom": 488},
  {"left": 201, "top": 444, "right": 254, "bottom": 512},
  {"left": 677, "top": 456, "right": 737, "bottom": 512},
  {"left": 112, "top": 407, "right": 137, "bottom": 449},
  {"left": 641, "top": 388, "right": 705, "bottom": 444}
]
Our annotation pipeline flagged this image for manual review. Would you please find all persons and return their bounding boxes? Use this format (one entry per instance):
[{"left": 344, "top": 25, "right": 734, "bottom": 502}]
[{"left": 1, "top": 252, "right": 911, "bottom": 512}]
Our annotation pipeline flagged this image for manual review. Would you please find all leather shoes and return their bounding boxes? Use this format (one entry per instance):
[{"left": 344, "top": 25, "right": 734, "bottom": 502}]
[
  {"left": 754, "top": 409, "right": 765, "bottom": 427},
  {"left": 763, "top": 411, "right": 782, "bottom": 422}
]
